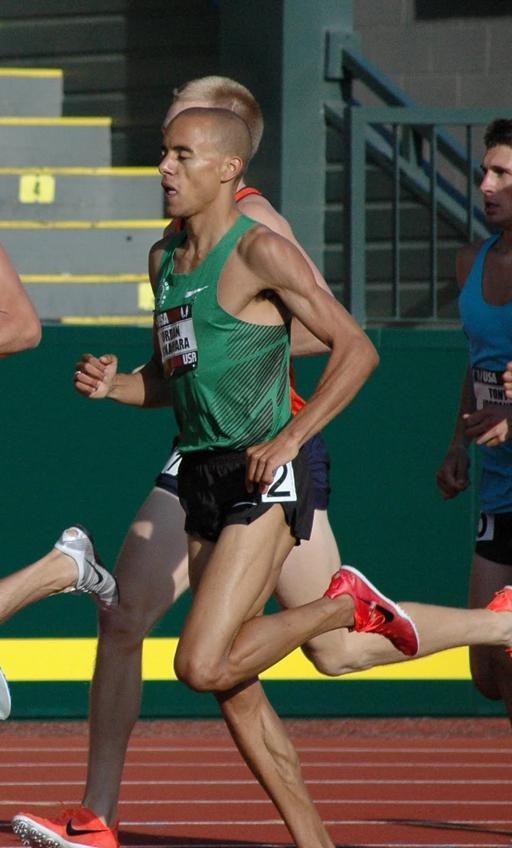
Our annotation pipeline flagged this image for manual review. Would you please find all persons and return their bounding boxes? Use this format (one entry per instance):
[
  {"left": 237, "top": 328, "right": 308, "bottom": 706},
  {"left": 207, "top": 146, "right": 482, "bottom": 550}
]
[
  {"left": 72, "top": 103, "right": 422, "bottom": 848},
  {"left": 437, "top": 116, "right": 511, "bottom": 704},
  {"left": 0, "top": 236, "right": 122, "bottom": 724},
  {"left": 9, "top": 73, "right": 511, "bottom": 848},
  {"left": 502, "top": 361, "right": 511, "bottom": 398}
]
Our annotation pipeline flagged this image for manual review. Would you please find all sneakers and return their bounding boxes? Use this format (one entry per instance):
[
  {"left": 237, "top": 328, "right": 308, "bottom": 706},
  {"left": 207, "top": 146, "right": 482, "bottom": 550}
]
[
  {"left": 12, "top": 805, "right": 120, "bottom": 848},
  {"left": 325, "top": 565, "right": 419, "bottom": 656},
  {"left": 487, "top": 584, "right": 512, "bottom": 657},
  {"left": 0, "top": 668, "right": 11, "bottom": 720},
  {"left": 54, "top": 524, "right": 122, "bottom": 611}
]
